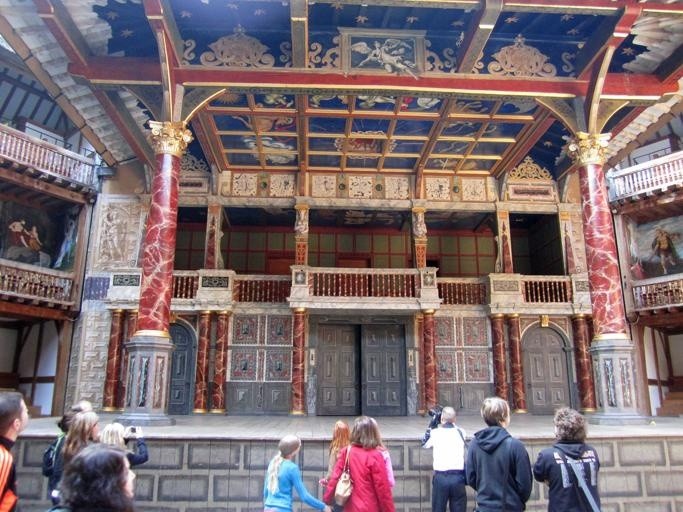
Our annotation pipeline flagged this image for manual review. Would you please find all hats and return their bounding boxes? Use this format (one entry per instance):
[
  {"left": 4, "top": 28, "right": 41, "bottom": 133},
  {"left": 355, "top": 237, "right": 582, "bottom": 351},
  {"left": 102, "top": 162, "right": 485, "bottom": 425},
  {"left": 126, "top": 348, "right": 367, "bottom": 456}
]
[{"left": 279, "top": 434, "right": 300, "bottom": 456}]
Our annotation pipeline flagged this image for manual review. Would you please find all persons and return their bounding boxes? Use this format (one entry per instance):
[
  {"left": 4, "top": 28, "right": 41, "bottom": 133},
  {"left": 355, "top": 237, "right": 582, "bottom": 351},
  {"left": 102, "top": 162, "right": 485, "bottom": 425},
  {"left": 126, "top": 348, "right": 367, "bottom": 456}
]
[
  {"left": 0, "top": 390, "right": 30, "bottom": 512},
  {"left": 46, "top": 441, "right": 136, "bottom": 511},
  {"left": 322, "top": 416, "right": 395, "bottom": 512},
  {"left": 466, "top": 395, "right": 532, "bottom": 512},
  {"left": 261, "top": 434, "right": 333, "bottom": 512},
  {"left": 317, "top": 418, "right": 352, "bottom": 487},
  {"left": 422, "top": 405, "right": 466, "bottom": 512},
  {"left": 47, "top": 410, "right": 100, "bottom": 505},
  {"left": 6, "top": 217, "right": 48, "bottom": 263},
  {"left": 370, "top": 419, "right": 395, "bottom": 494},
  {"left": 39, "top": 407, "right": 84, "bottom": 476},
  {"left": 532, "top": 407, "right": 601, "bottom": 512},
  {"left": 100, "top": 422, "right": 149, "bottom": 468},
  {"left": 71, "top": 399, "right": 93, "bottom": 413},
  {"left": 644, "top": 223, "right": 683, "bottom": 275}
]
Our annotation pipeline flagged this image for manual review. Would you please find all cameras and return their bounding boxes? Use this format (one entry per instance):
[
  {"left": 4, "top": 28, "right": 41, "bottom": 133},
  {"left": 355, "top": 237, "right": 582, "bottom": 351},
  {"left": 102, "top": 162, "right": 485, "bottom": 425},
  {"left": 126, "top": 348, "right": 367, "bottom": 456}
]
[
  {"left": 427, "top": 407, "right": 442, "bottom": 424},
  {"left": 130, "top": 427, "right": 136, "bottom": 433}
]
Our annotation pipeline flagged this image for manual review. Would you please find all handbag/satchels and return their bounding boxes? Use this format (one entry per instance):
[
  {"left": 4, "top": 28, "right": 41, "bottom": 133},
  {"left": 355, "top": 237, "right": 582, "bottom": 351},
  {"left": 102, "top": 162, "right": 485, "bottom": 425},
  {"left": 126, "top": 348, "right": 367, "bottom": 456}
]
[
  {"left": 42, "top": 434, "right": 65, "bottom": 476},
  {"left": 334, "top": 473, "right": 354, "bottom": 507}
]
